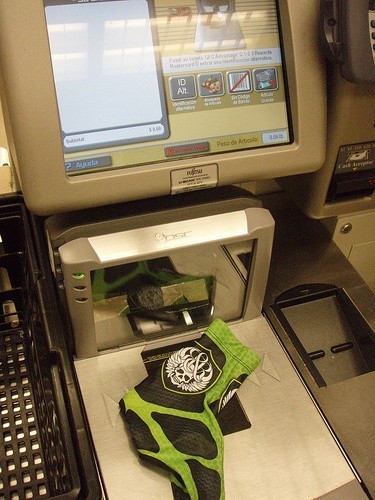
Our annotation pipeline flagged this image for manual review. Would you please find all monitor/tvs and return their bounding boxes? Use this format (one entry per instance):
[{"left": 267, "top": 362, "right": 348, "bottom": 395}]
[
  {"left": 58, "top": 205, "right": 275, "bottom": 358},
  {"left": 0, "top": 0, "right": 327, "bottom": 216}
]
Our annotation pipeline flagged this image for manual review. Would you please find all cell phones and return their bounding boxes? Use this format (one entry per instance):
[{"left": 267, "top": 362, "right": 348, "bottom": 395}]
[{"left": 128, "top": 275, "right": 210, "bottom": 314}]
